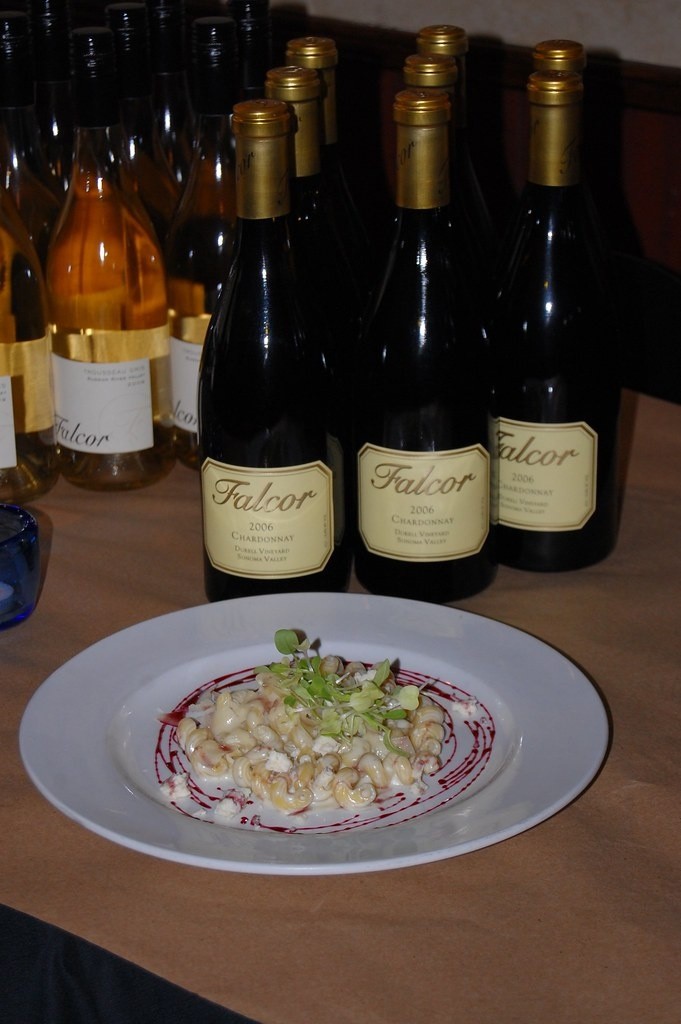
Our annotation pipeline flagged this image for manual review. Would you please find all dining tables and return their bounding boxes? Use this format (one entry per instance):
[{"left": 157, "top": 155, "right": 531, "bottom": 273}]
[{"left": 2, "top": 394, "right": 681, "bottom": 1024}]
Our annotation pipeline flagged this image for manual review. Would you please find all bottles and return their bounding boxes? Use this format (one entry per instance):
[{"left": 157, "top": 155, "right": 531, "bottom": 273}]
[
  {"left": 351, "top": 24, "right": 495, "bottom": 603},
  {"left": 1, "top": 0, "right": 272, "bottom": 504},
  {"left": 194, "top": 33, "right": 354, "bottom": 604},
  {"left": 489, "top": 40, "right": 618, "bottom": 572}
]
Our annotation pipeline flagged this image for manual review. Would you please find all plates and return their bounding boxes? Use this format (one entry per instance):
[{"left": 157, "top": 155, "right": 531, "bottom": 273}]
[{"left": 17, "top": 594, "right": 612, "bottom": 875}]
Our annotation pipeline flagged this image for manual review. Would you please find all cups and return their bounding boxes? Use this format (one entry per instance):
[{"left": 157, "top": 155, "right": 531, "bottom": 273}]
[{"left": 0, "top": 501, "right": 39, "bottom": 631}]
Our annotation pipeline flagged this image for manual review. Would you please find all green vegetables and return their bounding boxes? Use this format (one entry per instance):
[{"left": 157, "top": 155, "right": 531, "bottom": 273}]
[{"left": 253, "top": 628, "right": 430, "bottom": 760}]
